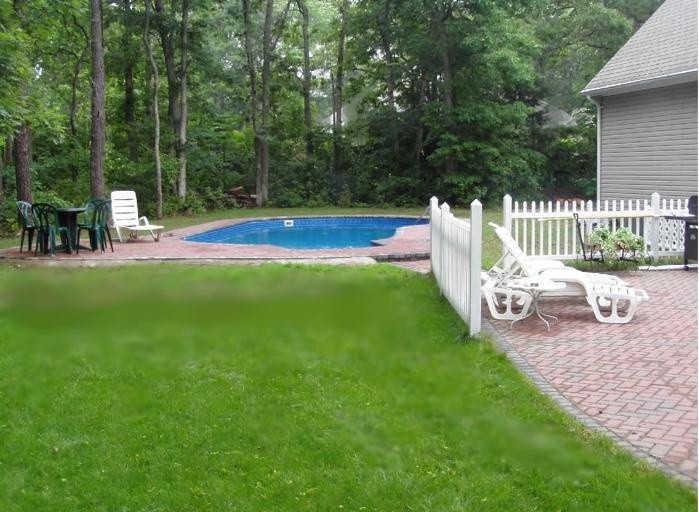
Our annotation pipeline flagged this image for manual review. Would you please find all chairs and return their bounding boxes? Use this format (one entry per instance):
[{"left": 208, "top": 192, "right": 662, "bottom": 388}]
[
  {"left": 481, "top": 222, "right": 652, "bottom": 323},
  {"left": 14, "top": 190, "right": 164, "bottom": 256}
]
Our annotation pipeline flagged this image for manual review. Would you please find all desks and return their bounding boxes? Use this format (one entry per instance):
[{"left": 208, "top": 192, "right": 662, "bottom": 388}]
[{"left": 505, "top": 279, "right": 567, "bottom": 331}]
